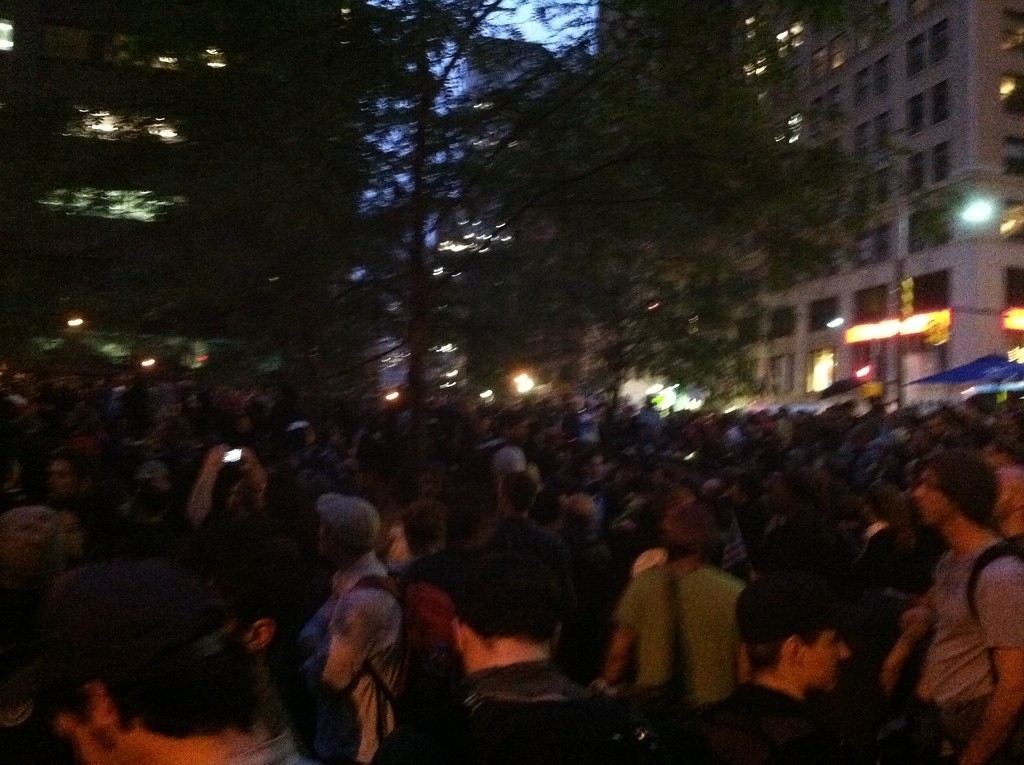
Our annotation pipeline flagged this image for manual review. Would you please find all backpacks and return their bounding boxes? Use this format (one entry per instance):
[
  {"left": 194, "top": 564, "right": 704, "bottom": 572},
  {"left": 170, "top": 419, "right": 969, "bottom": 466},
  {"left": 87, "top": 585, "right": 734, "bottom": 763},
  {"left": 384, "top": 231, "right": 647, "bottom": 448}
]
[{"left": 347, "top": 574, "right": 467, "bottom": 721}]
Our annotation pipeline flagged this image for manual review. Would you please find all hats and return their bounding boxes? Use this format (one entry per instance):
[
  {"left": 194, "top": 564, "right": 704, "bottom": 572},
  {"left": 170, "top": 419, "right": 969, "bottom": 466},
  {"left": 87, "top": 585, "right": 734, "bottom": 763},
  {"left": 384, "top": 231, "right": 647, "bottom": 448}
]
[
  {"left": 662, "top": 495, "right": 729, "bottom": 542},
  {"left": 316, "top": 491, "right": 380, "bottom": 538},
  {"left": 495, "top": 447, "right": 527, "bottom": 472},
  {"left": 0, "top": 505, "right": 67, "bottom": 579}
]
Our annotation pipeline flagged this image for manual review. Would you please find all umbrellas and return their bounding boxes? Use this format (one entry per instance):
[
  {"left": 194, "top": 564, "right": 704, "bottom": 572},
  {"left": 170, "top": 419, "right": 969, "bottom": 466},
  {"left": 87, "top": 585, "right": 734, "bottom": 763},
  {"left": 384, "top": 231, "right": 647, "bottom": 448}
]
[
  {"left": 906, "top": 354, "right": 1024, "bottom": 387},
  {"left": 822, "top": 377, "right": 864, "bottom": 399}
]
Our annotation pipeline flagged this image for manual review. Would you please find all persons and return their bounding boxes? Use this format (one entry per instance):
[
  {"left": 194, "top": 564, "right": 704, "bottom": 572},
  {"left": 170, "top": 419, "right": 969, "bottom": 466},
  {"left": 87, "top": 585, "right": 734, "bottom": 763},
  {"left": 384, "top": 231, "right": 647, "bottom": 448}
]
[{"left": 0, "top": 361, "right": 1024, "bottom": 765}]
